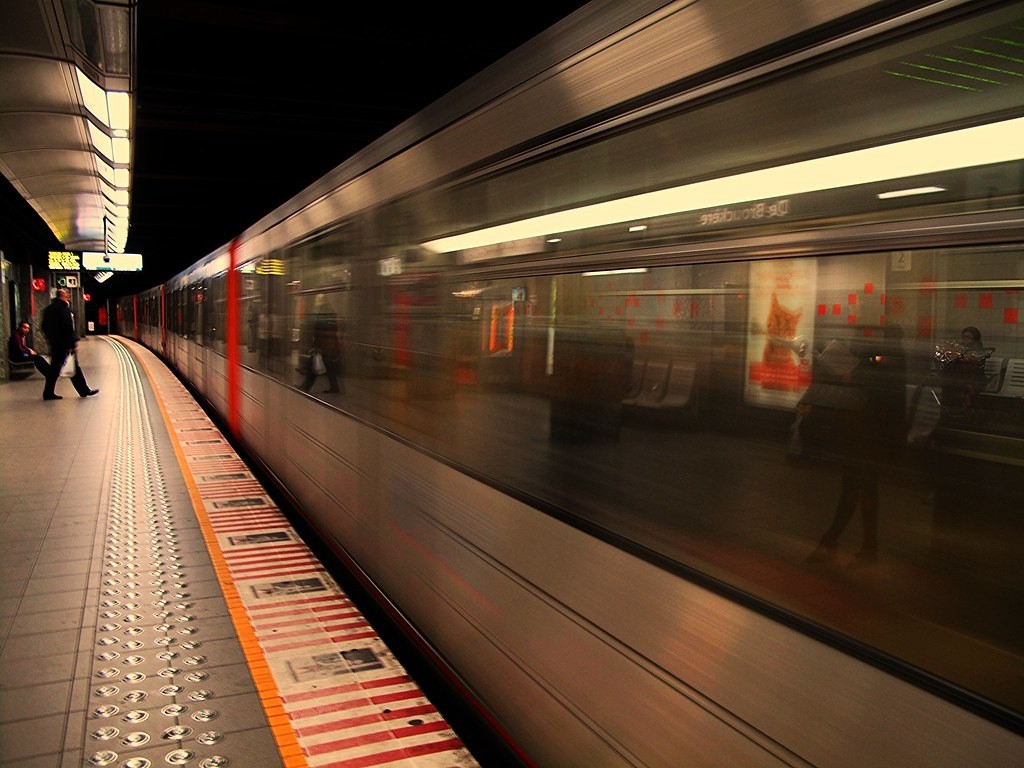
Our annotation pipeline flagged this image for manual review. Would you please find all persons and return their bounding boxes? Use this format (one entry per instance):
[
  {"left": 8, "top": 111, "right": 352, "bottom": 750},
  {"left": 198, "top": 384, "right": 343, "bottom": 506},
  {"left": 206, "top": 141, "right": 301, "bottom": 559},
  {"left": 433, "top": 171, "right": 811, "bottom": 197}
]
[
  {"left": 246, "top": 293, "right": 991, "bottom": 570},
  {"left": 8, "top": 322, "right": 50, "bottom": 377},
  {"left": 41, "top": 290, "right": 99, "bottom": 400}
]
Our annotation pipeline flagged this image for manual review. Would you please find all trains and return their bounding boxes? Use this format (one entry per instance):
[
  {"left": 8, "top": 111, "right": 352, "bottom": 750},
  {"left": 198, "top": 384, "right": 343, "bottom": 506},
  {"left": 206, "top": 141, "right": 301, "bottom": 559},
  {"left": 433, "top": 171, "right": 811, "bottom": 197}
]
[{"left": 127, "top": 0, "right": 1023, "bottom": 766}]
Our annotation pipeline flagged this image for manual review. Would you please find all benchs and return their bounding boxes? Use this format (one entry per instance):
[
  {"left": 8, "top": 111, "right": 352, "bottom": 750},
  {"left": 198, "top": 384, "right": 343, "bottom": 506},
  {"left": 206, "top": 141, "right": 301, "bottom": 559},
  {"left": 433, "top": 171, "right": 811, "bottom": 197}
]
[{"left": 7, "top": 340, "right": 47, "bottom": 381}]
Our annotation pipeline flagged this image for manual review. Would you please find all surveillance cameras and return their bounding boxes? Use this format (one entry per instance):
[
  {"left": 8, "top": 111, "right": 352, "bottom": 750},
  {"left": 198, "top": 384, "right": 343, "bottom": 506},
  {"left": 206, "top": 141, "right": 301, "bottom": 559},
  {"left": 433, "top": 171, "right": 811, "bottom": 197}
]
[{"left": 104, "top": 256, "right": 110, "bottom": 263}]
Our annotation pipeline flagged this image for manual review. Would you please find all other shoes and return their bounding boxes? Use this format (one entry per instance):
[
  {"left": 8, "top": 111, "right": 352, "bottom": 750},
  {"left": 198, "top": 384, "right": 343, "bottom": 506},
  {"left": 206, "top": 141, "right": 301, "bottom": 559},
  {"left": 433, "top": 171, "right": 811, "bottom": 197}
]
[
  {"left": 81, "top": 390, "right": 99, "bottom": 397},
  {"left": 324, "top": 386, "right": 340, "bottom": 394},
  {"left": 805, "top": 542, "right": 838, "bottom": 563},
  {"left": 292, "top": 385, "right": 309, "bottom": 393},
  {"left": 293, "top": 368, "right": 308, "bottom": 375},
  {"left": 44, "top": 395, "right": 63, "bottom": 400}
]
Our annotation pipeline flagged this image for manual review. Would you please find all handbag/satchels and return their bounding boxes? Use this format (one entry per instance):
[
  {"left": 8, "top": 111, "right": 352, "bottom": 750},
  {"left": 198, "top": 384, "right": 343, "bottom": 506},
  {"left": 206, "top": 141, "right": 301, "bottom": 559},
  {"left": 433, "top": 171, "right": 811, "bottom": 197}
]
[
  {"left": 59, "top": 352, "right": 76, "bottom": 378},
  {"left": 314, "top": 352, "right": 328, "bottom": 376}
]
